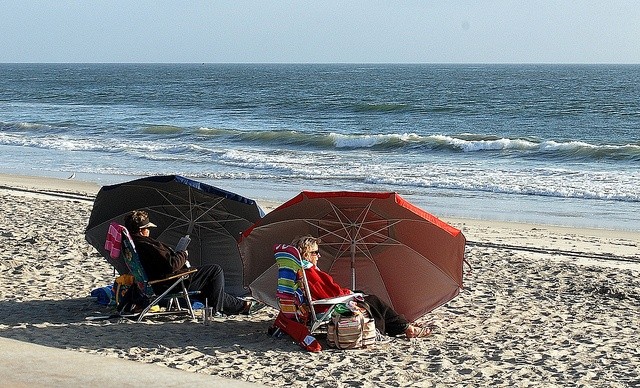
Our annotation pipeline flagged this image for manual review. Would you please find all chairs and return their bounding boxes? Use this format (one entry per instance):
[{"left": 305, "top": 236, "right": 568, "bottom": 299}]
[
  {"left": 273, "top": 244, "right": 368, "bottom": 336},
  {"left": 121, "top": 230, "right": 201, "bottom": 321}
]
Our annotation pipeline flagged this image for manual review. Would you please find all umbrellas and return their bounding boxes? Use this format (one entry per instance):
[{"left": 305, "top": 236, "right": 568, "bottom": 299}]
[
  {"left": 85, "top": 174, "right": 266, "bottom": 299},
  {"left": 236, "top": 190, "right": 466, "bottom": 325}
]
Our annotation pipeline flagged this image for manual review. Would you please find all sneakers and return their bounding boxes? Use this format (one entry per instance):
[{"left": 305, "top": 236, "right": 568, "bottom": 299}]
[
  {"left": 250, "top": 302, "right": 267, "bottom": 315},
  {"left": 214, "top": 311, "right": 235, "bottom": 319}
]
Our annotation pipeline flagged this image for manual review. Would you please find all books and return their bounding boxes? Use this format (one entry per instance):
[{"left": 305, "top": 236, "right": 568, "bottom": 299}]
[
  {"left": 339, "top": 306, "right": 373, "bottom": 325},
  {"left": 174, "top": 234, "right": 191, "bottom": 251}
]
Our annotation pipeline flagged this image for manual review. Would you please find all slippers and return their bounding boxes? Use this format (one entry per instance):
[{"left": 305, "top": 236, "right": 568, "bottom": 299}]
[{"left": 408, "top": 327, "right": 431, "bottom": 336}]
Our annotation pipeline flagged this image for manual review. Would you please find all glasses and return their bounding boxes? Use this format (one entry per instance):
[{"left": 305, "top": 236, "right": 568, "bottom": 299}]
[{"left": 308, "top": 249, "right": 319, "bottom": 255}]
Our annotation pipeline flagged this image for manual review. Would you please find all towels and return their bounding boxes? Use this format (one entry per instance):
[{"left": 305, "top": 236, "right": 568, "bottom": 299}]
[{"left": 103, "top": 222, "right": 135, "bottom": 259}]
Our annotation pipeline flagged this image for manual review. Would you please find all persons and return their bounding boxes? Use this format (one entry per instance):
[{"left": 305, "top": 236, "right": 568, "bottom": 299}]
[
  {"left": 124, "top": 212, "right": 268, "bottom": 314},
  {"left": 291, "top": 233, "right": 431, "bottom": 337}
]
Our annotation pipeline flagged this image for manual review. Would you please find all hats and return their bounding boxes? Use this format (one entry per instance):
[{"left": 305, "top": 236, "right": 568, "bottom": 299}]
[{"left": 124, "top": 211, "right": 158, "bottom": 228}]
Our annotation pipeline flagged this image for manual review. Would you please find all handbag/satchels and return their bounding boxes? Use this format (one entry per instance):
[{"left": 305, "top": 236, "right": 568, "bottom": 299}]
[{"left": 326, "top": 313, "right": 377, "bottom": 349}]
